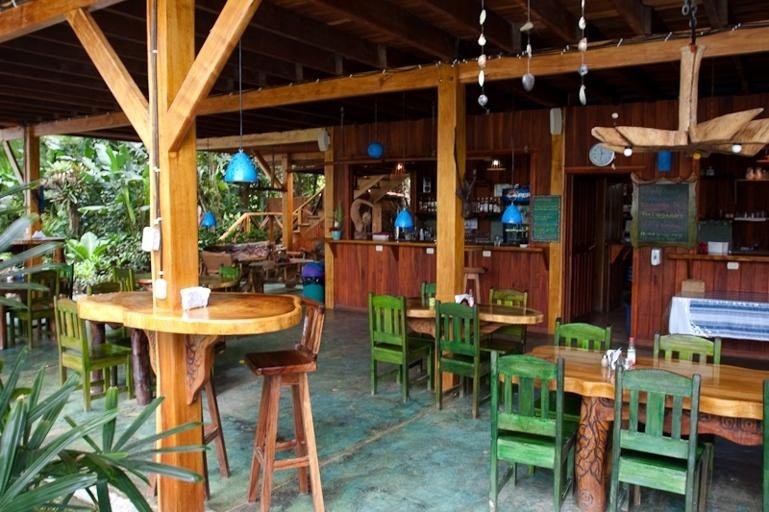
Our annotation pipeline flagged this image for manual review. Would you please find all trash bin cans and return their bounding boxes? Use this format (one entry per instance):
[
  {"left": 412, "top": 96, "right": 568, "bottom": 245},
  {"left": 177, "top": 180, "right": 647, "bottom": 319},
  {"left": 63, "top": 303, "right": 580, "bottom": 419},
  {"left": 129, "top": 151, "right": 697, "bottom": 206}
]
[
  {"left": 625, "top": 297, "right": 632, "bottom": 335},
  {"left": 303, "top": 276, "right": 326, "bottom": 305}
]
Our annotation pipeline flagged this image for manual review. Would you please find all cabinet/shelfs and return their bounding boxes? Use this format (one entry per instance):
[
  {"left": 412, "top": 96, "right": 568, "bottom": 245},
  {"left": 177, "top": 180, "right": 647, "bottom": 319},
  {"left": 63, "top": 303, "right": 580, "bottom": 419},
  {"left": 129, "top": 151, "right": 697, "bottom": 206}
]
[{"left": 351, "top": 156, "right": 530, "bottom": 244}]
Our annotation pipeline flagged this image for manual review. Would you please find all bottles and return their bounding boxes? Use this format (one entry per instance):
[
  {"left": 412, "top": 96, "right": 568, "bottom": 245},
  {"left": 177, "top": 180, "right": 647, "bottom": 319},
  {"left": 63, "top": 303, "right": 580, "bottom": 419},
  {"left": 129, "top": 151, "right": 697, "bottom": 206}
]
[
  {"left": 602, "top": 368, "right": 612, "bottom": 379},
  {"left": 625, "top": 336, "right": 637, "bottom": 371},
  {"left": 600, "top": 355, "right": 611, "bottom": 367},
  {"left": 471, "top": 196, "right": 501, "bottom": 214},
  {"left": 744, "top": 210, "right": 765, "bottom": 218},
  {"left": 508, "top": 206, "right": 529, "bottom": 242},
  {"left": 414, "top": 224, "right": 432, "bottom": 241},
  {"left": 154, "top": 271, "right": 167, "bottom": 300},
  {"left": 698, "top": 241, "right": 707, "bottom": 255},
  {"left": 467, "top": 288, "right": 474, "bottom": 306},
  {"left": 701, "top": 166, "right": 715, "bottom": 175},
  {"left": 417, "top": 195, "right": 437, "bottom": 211}
]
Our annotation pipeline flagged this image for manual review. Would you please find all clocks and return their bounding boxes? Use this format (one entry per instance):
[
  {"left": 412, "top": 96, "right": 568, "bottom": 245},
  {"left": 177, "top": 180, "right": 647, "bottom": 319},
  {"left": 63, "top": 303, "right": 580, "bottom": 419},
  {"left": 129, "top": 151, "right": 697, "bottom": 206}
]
[{"left": 589, "top": 142, "right": 615, "bottom": 167}]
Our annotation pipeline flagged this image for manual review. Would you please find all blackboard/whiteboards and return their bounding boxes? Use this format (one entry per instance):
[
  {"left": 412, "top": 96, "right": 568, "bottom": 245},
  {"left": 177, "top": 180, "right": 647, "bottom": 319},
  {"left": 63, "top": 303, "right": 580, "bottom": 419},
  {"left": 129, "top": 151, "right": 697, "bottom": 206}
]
[
  {"left": 632, "top": 182, "right": 696, "bottom": 247},
  {"left": 531, "top": 195, "right": 561, "bottom": 243}
]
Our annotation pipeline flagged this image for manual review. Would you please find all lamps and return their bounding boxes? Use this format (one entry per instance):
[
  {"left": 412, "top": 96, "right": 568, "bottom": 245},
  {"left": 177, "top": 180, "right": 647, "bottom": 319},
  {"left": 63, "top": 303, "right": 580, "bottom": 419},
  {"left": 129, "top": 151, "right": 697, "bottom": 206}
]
[
  {"left": 200, "top": 40, "right": 259, "bottom": 229},
  {"left": 500, "top": 110, "right": 523, "bottom": 225},
  {"left": 393, "top": 92, "right": 415, "bottom": 229}
]
